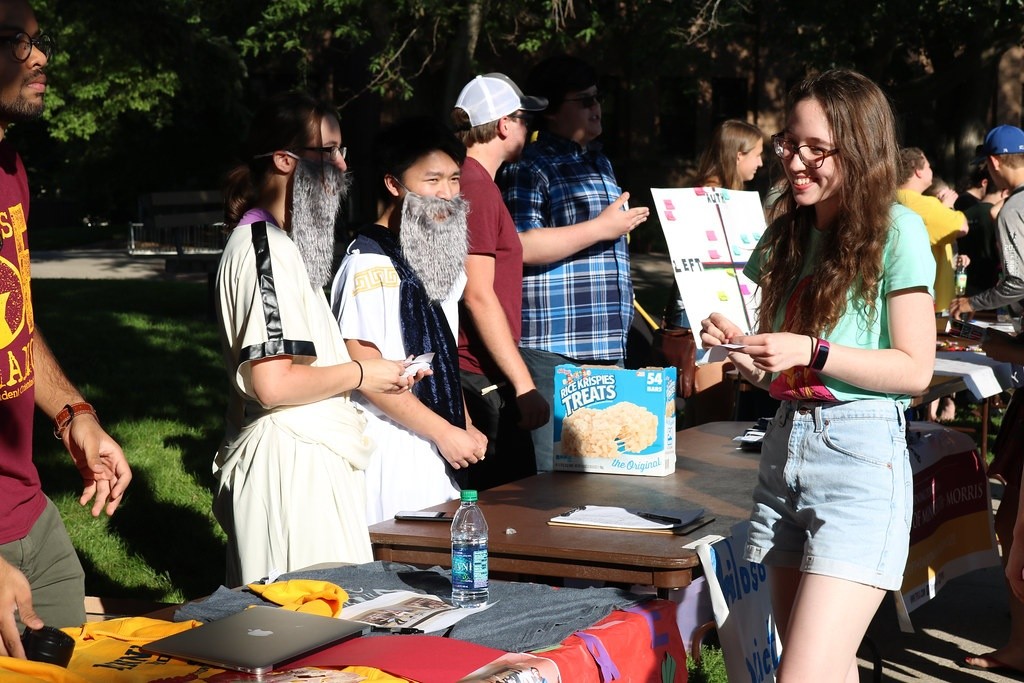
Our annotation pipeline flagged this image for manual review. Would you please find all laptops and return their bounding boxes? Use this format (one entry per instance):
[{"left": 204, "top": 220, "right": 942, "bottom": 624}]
[{"left": 142, "top": 605, "right": 373, "bottom": 673}]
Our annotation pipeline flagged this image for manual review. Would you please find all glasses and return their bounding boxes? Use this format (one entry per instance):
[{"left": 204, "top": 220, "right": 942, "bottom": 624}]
[
  {"left": 0, "top": 32, "right": 52, "bottom": 62},
  {"left": 565, "top": 90, "right": 606, "bottom": 108},
  {"left": 771, "top": 129, "right": 839, "bottom": 169},
  {"left": 286, "top": 145, "right": 348, "bottom": 161}
]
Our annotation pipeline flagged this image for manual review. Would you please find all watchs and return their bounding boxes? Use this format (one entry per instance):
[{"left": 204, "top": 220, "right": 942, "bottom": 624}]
[{"left": 805, "top": 337, "right": 830, "bottom": 372}]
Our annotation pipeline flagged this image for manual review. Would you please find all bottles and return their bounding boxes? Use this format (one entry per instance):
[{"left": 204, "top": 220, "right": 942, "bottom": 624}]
[
  {"left": 996, "top": 273, "right": 1009, "bottom": 322},
  {"left": 451, "top": 490, "right": 490, "bottom": 609},
  {"left": 955, "top": 258, "right": 967, "bottom": 295}
]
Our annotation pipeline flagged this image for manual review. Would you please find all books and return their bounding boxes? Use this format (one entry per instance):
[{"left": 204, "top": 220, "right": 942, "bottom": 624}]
[{"left": 548, "top": 504, "right": 717, "bottom": 535}]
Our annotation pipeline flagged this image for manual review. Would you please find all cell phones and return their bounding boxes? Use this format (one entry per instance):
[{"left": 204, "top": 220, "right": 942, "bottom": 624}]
[{"left": 394, "top": 510, "right": 454, "bottom": 522}]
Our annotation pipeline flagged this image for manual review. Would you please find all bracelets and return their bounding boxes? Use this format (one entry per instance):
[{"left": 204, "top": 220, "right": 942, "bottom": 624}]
[
  {"left": 351, "top": 359, "right": 363, "bottom": 388},
  {"left": 52, "top": 402, "right": 100, "bottom": 439}
]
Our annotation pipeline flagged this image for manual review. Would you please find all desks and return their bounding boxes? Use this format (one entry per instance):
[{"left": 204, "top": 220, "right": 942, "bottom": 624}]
[
  {"left": 0, "top": 562, "right": 690, "bottom": 683},
  {"left": 906, "top": 353, "right": 1011, "bottom": 490},
  {"left": 369, "top": 422, "right": 768, "bottom": 601}
]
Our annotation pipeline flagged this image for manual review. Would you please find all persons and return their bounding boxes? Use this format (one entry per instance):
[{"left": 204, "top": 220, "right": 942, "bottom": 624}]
[
  {"left": 492, "top": 55, "right": 649, "bottom": 475},
  {"left": 699, "top": 68, "right": 935, "bottom": 683},
  {"left": 0, "top": 0, "right": 132, "bottom": 662},
  {"left": 211, "top": 92, "right": 433, "bottom": 587},
  {"left": 331, "top": 116, "right": 488, "bottom": 524},
  {"left": 455, "top": 71, "right": 550, "bottom": 491},
  {"left": 661, "top": 118, "right": 1024, "bottom": 670}
]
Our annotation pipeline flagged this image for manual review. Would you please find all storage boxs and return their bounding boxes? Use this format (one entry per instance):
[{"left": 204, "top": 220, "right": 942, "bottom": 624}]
[{"left": 549, "top": 365, "right": 678, "bottom": 478}]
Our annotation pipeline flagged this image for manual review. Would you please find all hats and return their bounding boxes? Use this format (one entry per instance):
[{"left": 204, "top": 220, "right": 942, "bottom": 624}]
[
  {"left": 970, "top": 123, "right": 1024, "bottom": 166},
  {"left": 524, "top": 54, "right": 619, "bottom": 101},
  {"left": 455, "top": 72, "right": 550, "bottom": 127}
]
